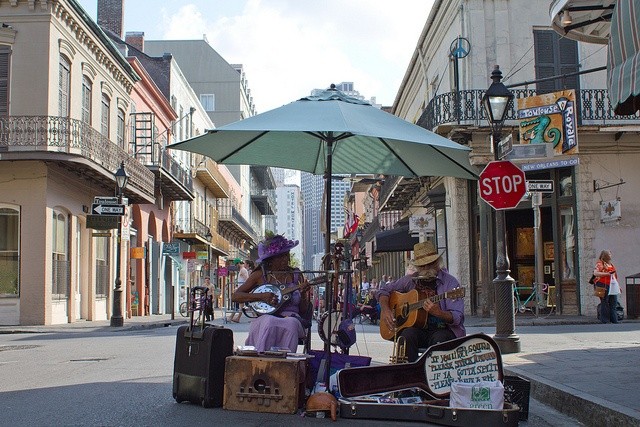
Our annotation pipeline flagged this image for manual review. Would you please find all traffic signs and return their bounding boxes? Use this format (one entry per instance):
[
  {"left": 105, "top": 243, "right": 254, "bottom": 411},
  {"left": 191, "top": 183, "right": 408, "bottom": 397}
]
[
  {"left": 479, "top": 65, "right": 525, "bottom": 353},
  {"left": 91, "top": 204, "right": 125, "bottom": 215},
  {"left": 525, "top": 180, "right": 554, "bottom": 193}
]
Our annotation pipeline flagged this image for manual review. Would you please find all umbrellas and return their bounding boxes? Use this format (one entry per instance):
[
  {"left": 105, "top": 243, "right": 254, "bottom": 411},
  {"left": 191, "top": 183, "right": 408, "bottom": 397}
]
[{"left": 164, "top": 83, "right": 481, "bottom": 400}]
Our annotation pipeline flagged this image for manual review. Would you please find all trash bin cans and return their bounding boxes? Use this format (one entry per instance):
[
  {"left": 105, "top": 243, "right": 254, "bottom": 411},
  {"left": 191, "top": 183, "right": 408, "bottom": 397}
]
[{"left": 625, "top": 273, "right": 640, "bottom": 319}]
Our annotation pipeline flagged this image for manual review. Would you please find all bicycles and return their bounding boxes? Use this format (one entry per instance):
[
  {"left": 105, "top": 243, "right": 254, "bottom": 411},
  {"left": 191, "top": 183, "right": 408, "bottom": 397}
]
[{"left": 510, "top": 280, "right": 555, "bottom": 321}]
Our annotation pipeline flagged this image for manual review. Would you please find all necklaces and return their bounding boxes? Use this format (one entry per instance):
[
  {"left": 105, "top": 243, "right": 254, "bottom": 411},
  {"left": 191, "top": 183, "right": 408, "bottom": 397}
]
[{"left": 270, "top": 270, "right": 288, "bottom": 284}]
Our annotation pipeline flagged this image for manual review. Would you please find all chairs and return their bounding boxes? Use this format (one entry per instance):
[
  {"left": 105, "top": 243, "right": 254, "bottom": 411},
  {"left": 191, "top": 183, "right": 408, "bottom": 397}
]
[
  {"left": 297, "top": 302, "right": 313, "bottom": 354},
  {"left": 353, "top": 300, "right": 377, "bottom": 325}
]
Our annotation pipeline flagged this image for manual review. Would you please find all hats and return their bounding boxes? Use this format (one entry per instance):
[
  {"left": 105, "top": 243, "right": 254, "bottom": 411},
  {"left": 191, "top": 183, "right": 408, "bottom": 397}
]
[
  {"left": 252, "top": 235, "right": 298, "bottom": 271},
  {"left": 411, "top": 241, "right": 445, "bottom": 266},
  {"left": 233, "top": 258, "right": 241, "bottom": 265}
]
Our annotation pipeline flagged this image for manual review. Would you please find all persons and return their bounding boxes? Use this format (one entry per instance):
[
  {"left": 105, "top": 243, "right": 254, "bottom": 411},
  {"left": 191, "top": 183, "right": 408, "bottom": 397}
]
[
  {"left": 322, "top": 291, "right": 326, "bottom": 299},
  {"left": 360, "top": 290, "right": 377, "bottom": 323},
  {"left": 594, "top": 248, "right": 623, "bottom": 323},
  {"left": 234, "top": 284, "right": 238, "bottom": 289},
  {"left": 379, "top": 274, "right": 387, "bottom": 289},
  {"left": 386, "top": 276, "right": 394, "bottom": 284},
  {"left": 226, "top": 258, "right": 249, "bottom": 321},
  {"left": 231, "top": 229, "right": 311, "bottom": 352},
  {"left": 378, "top": 240, "right": 466, "bottom": 362},
  {"left": 201, "top": 276, "right": 215, "bottom": 322},
  {"left": 315, "top": 293, "right": 318, "bottom": 310},
  {"left": 369, "top": 278, "right": 379, "bottom": 288},
  {"left": 359, "top": 275, "right": 369, "bottom": 289},
  {"left": 145, "top": 284, "right": 149, "bottom": 315},
  {"left": 232, "top": 259, "right": 255, "bottom": 322}
]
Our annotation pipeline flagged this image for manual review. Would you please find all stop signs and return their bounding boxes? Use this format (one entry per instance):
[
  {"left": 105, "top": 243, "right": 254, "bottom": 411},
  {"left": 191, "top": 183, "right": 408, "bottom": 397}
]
[{"left": 479, "top": 160, "right": 527, "bottom": 211}]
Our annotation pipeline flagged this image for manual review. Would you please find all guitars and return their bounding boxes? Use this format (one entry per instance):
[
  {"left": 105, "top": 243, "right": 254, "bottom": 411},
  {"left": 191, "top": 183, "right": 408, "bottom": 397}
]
[{"left": 380, "top": 286, "right": 465, "bottom": 342}]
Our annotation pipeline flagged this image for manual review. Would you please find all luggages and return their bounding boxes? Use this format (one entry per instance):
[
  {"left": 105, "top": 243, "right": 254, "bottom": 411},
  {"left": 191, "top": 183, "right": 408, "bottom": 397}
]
[{"left": 172, "top": 286, "right": 233, "bottom": 408}]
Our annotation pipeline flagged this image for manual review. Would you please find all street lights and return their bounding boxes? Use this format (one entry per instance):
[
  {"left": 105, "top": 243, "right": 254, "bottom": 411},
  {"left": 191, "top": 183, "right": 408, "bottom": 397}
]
[
  {"left": 111, "top": 159, "right": 130, "bottom": 327},
  {"left": 205, "top": 228, "right": 214, "bottom": 321}
]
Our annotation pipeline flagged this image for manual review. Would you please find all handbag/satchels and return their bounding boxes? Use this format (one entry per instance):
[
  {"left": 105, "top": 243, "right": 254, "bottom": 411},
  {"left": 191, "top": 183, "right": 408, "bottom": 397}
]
[
  {"left": 339, "top": 274, "right": 356, "bottom": 346},
  {"left": 306, "top": 350, "right": 371, "bottom": 398},
  {"left": 594, "top": 261, "right": 607, "bottom": 298}
]
